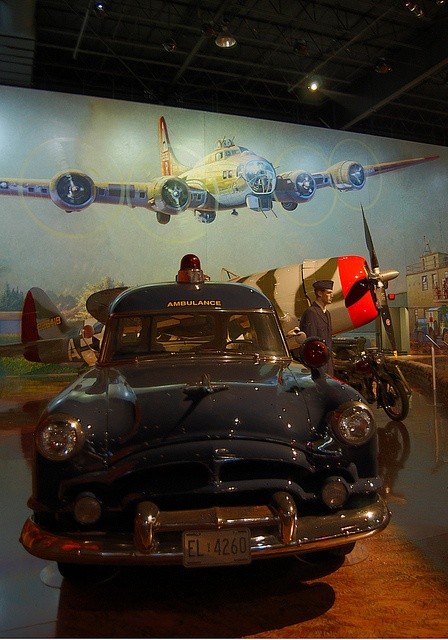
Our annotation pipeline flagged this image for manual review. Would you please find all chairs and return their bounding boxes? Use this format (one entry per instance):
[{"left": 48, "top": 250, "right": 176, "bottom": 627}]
[{"left": 225, "top": 314, "right": 257, "bottom": 351}]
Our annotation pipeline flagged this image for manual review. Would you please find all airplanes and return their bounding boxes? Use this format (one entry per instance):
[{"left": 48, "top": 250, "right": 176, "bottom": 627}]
[
  {"left": 0, "top": 115, "right": 441, "bottom": 244},
  {"left": 20, "top": 202, "right": 400, "bottom": 377}
]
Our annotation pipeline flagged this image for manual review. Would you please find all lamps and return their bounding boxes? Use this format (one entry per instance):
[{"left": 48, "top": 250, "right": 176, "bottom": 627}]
[
  {"left": 214, "top": 16, "right": 237, "bottom": 50},
  {"left": 372, "top": 55, "right": 391, "bottom": 74}
]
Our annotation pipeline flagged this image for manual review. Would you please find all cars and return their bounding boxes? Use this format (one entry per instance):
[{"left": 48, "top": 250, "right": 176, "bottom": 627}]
[{"left": 17, "top": 253, "right": 390, "bottom": 590}]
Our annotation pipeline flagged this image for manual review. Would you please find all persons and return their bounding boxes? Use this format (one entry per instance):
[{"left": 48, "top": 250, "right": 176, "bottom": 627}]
[{"left": 299, "top": 280, "right": 337, "bottom": 376}]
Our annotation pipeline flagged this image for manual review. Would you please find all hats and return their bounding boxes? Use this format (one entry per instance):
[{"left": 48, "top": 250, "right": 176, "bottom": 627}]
[{"left": 313, "top": 280, "right": 332, "bottom": 290}]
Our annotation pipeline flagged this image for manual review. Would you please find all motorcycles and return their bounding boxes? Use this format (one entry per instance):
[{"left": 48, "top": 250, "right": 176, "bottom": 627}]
[
  {"left": 377, "top": 421, "right": 410, "bottom": 506},
  {"left": 331, "top": 350, "right": 409, "bottom": 422}
]
